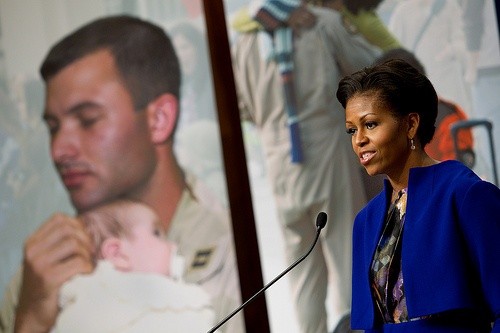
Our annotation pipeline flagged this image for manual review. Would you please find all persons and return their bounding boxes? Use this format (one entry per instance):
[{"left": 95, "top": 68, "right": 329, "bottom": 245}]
[
  {"left": 245, "top": 0, "right": 401, "bottom": 55},
  {"left": 0, "top": 15, "right": 245, "bottom": 333},
  {"left": 49, "top": 199, "right": 221, "bottom": 333},
  {"left": 336, "top": 59, "right": 500, "bottom": 333},
  {"left": 369, "top": 47, "right": 472, "bottom": 164},
  {"left": 231, "top": 0, "right": 385, "bottom": 333}
]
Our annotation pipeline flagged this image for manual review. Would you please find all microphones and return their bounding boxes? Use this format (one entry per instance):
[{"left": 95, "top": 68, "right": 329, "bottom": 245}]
[{"left": 207, "top": 212, "right": 328, "bottom": 333}]
